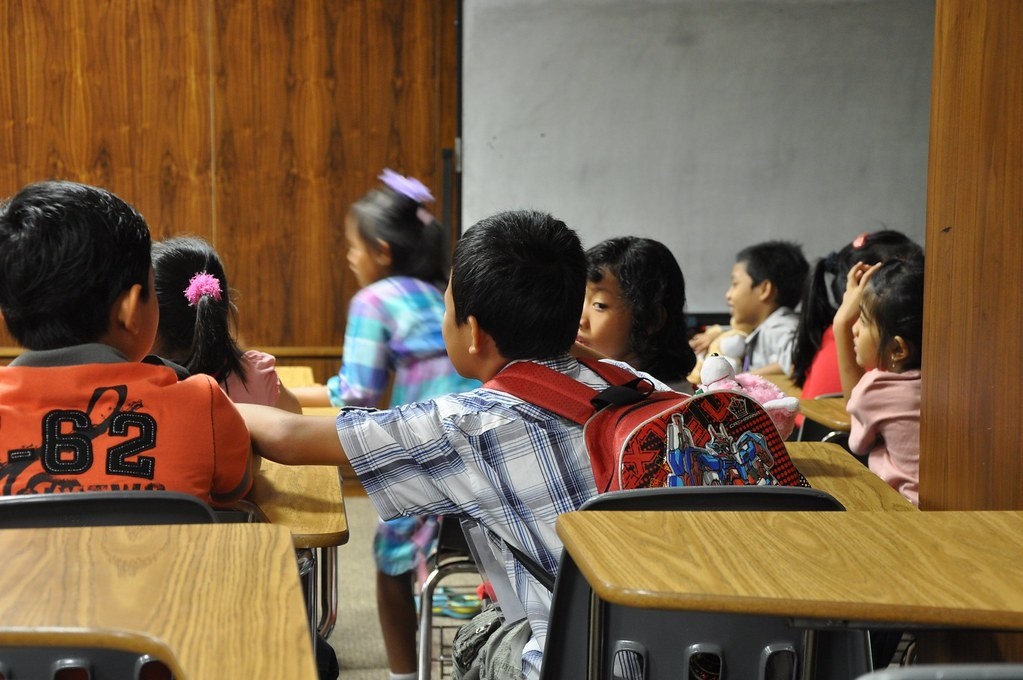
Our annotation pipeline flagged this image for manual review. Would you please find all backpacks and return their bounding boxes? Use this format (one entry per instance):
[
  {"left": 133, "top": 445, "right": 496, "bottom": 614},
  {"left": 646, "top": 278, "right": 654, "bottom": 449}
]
[{"left": 484, "top": 344, "right": 812, "bottom": 496}]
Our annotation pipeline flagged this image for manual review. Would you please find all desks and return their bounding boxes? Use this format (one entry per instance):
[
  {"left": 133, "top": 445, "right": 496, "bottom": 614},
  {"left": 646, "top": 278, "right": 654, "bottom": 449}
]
[
  {"left": 418, "top": 374, "right": 1023, "bottom": 680},
  {"left": 0, "top": 366, "right": 348, "bottom": 680}
]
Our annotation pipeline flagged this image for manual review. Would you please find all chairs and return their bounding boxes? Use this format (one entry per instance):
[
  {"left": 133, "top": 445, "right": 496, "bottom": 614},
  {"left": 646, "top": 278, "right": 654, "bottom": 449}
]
[
  {"left": 798, "top": 393, "right": 845, "bottom": 442},
  {"left": 1, "top": 490, "right": 220, "bottom": 680},
  {"left": 542, "top": 485, "right": 874, "bottom": 680}
]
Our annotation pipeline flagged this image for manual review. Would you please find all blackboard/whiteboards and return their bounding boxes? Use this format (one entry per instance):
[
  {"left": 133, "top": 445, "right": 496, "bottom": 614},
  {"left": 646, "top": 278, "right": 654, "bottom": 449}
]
[{"left": 452, "top": 0, "right": 936, "bottom": 323}]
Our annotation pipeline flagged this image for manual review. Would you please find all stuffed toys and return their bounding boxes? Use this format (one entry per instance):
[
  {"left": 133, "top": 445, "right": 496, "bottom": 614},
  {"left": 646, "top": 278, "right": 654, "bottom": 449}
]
[{"left": 694, "top": 352, "right": 798, "bottom": 439}]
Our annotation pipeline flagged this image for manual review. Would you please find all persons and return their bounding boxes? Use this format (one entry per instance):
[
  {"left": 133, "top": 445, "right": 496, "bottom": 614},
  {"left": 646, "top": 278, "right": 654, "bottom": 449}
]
[
  {"left": 290, "top": 169, "right": 482, "bottom": 680},
  {"left": 234, "top": 209, "right": 678, "bottom": 680},
  {"left": 147, "top": 236, "right": 304, "bottom": 415},
  {"left": 688, "top": 228, "right": 926, "bottom": 510},
  {"left": 0, "top": 181, "right": 340, "bottom": 680},
  {"left": 568, "top": 236, "right": 695, "bottom": 396}
]
